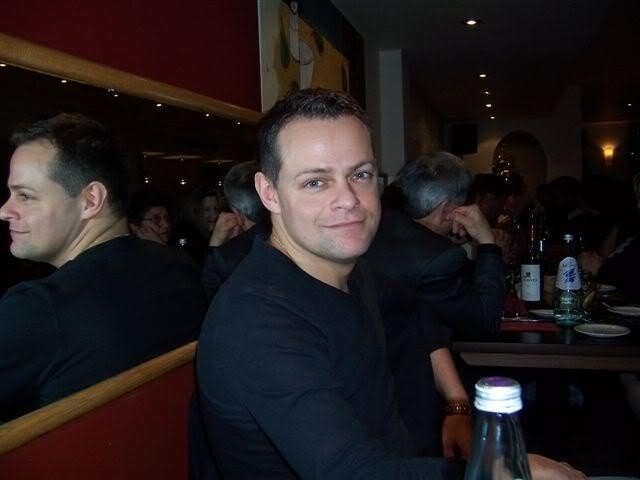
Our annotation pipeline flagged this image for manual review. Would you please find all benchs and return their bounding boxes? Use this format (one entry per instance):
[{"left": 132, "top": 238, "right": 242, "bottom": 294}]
[{"left": 0, "top": 340, "right": 202, "bottom": 479}]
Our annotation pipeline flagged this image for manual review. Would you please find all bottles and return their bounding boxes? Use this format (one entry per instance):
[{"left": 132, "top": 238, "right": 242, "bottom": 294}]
[
  {"left": 555, "top": 233, "right": 585, "bottom": 327},
  {"left": 519, "top": 211, "right": 543, "bottom": 309},
  {"left": 465, "top": 374, "right": 535, "bottom": 479}
]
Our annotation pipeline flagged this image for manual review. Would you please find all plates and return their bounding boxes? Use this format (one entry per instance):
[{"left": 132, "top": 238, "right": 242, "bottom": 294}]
[
  {"left": 526, "top": 310, "right": 555, "bottom": 318},
  {"left": 607, "top": 304, "right": 640, "bottom": 316},
  {"left": 577, "top": 322, "right": 631, "bottom": 341},
  {"left": 595, "top": 283, "right": 615, "bottom": 294}
]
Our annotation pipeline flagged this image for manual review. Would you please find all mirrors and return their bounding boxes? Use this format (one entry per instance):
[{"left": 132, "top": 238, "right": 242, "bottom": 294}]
[{"left": 0, "top": 34, "right": 269, "bottom": 453}]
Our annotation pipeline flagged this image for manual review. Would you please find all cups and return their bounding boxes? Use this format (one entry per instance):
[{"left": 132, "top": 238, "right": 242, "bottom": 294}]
[{"left": 544, "top": 275, "right": 557, "bottom": 293}]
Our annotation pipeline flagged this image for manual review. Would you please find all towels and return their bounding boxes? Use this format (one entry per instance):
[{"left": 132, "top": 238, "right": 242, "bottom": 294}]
[{"left": 501, "top": 295, "right": 562, "bottom": 332}]
